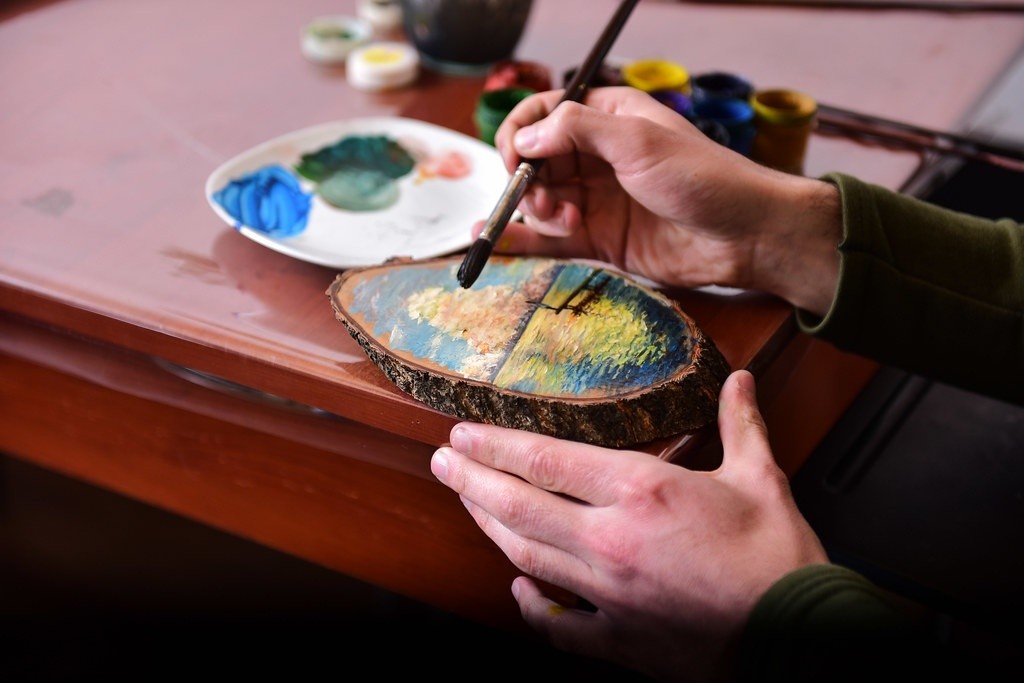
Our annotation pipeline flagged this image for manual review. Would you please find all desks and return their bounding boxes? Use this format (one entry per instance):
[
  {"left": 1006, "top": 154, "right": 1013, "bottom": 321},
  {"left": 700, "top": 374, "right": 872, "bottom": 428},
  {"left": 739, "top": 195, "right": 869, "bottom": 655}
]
[{"left": 0, "top": 0, "right": 1024, "bottom": 640}]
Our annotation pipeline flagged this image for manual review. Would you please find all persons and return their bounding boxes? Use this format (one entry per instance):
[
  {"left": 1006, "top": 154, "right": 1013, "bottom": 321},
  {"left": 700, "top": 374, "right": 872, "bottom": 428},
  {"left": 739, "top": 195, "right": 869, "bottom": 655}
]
[{"left": 428, "top": 87, "right": 1024, "bottom": 682}]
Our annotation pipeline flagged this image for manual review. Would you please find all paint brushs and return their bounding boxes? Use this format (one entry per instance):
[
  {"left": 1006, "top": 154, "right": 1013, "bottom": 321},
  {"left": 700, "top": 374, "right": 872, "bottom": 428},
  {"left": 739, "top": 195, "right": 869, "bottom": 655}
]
[{"left": 456, "top": 0, "right": 642, "bottom": 289}]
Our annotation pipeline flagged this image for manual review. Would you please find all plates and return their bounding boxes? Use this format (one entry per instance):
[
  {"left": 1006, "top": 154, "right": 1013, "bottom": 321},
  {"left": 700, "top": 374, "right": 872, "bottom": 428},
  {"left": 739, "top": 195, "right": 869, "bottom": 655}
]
[{"left": 204, "top": 116, "right": 522, "bottom": 270}]
